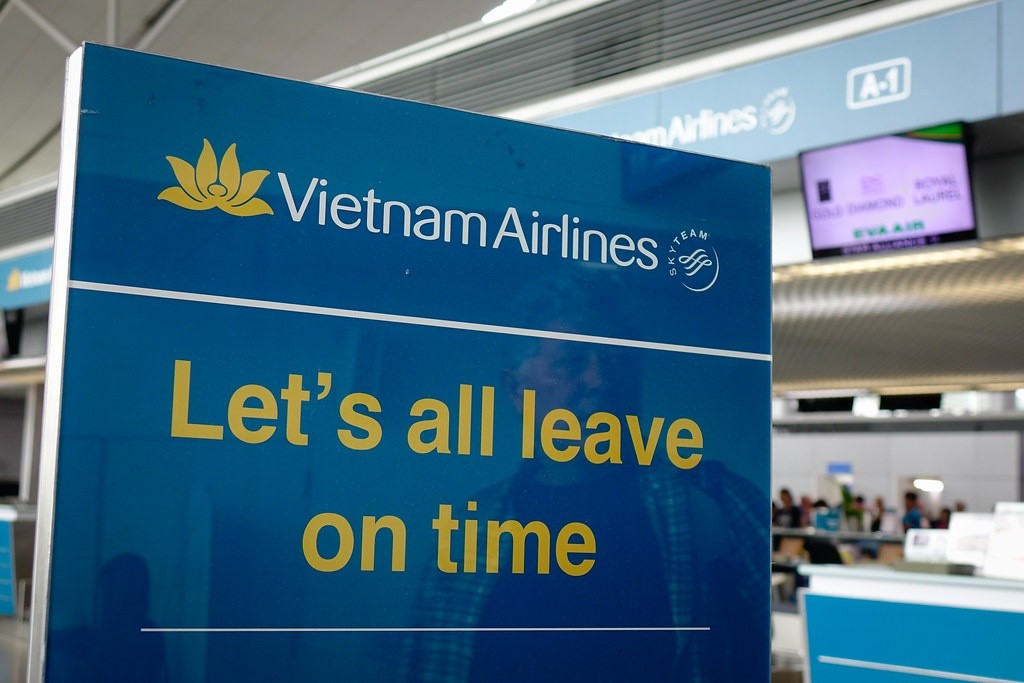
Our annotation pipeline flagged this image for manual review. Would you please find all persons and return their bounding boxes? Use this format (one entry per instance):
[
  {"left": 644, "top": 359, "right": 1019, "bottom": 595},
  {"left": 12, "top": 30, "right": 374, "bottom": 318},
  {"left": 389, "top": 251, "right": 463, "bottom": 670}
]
[{"left": 772, "top": 481, "right": 970, "bottom": 595}]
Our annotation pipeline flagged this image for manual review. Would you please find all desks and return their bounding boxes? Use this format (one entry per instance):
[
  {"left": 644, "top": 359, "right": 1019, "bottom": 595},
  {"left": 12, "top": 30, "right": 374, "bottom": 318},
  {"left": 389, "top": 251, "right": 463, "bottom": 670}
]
[{"left": 772, "top": 527, "right": 904, "bottom": 564}]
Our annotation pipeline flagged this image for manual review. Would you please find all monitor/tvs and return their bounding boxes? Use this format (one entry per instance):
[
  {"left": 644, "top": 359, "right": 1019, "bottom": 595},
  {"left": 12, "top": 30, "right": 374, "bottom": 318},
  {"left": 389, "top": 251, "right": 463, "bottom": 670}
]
[{"left": 798, "top": 121, "right": 980, "bottom": 263}]
